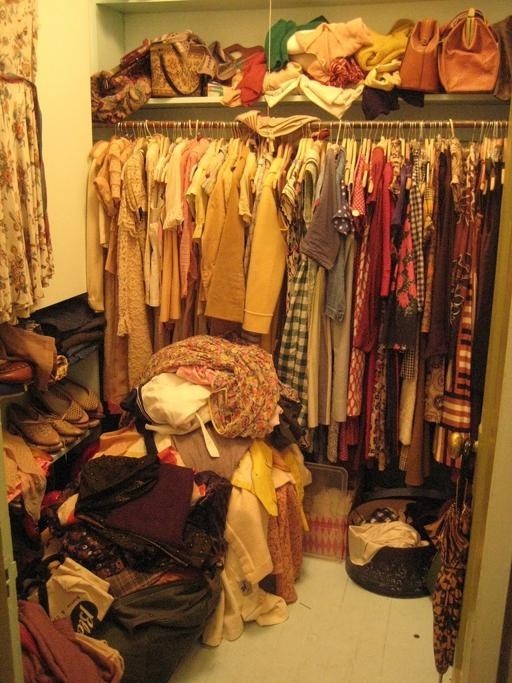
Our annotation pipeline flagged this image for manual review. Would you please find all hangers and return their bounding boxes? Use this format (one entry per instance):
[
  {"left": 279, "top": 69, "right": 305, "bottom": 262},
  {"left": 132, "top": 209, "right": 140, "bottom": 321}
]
[{"left": 110, "top": 118, "right": 512, "bottom": 218}]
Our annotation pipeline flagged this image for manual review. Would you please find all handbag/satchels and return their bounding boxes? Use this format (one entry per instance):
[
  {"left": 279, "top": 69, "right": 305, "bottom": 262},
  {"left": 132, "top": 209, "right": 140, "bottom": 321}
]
[
  {"left": 18, "top": 455, "right": 232, "bottom": 682},
  {"left": 400, "top": 8, "right": 512, "bottom": 101},
  {"left": 91, "top": 30, "right": 264, "bottom": 124}
]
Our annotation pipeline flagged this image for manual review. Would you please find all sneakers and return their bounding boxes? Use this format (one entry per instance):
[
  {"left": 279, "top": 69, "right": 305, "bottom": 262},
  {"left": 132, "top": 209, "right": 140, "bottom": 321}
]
[{"left": 4, "top": 375, "right": 103, "bottom": 453}]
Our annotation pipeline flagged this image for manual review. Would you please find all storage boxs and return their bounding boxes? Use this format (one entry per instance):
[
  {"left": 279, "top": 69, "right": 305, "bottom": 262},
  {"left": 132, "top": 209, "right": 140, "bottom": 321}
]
[{"left": 298, "top": 458, "right": 361, "bottom": 562}]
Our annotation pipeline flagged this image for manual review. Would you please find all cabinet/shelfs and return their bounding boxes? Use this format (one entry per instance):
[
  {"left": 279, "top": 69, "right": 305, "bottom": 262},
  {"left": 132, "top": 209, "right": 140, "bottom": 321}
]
[
  {"left": 92, "top": 0, "right": 512, "bottom": 107},
  {"left": 0, "top": 292, "right": 107, "bottom": 505}
]
